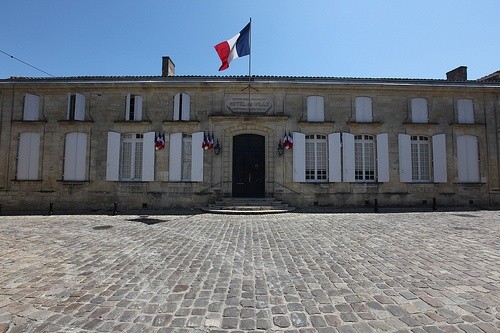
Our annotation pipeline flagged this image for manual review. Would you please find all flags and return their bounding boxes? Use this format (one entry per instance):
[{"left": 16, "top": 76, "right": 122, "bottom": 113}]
[
  {"left": 283, "top": 132, "right": 288, "bottom": 149},
  {"left": 288, "top": 132, "right": 293, "bottom": 149},
  {"left": 213, "top": 22, "right": 250, "bottom": 71},
  {"left": 154, "top": 130, "right": 214, "bottom": 152}
]
